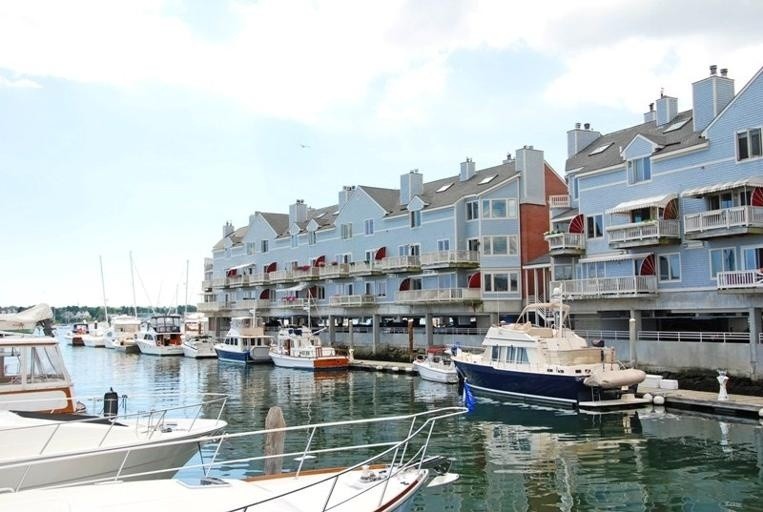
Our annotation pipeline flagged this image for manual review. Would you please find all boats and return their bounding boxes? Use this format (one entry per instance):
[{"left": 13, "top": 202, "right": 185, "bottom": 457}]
[
  {"left": 0, "top": 388, "right": 233, "bottom": 494},
  {"left": 0, "top": 302, "right": 87, "bottom": 415},
  {"left": 214, "top": 300, "right": 271, "bottom": 364},
  {"left": 448, "top": 284, "right": 653, "bottom": 413},
  {"left": 268, "top": 287, "right": 348, "bottom": 371},
  {"left": 0, "top": 404, "right": 470, "bottom": 512},
  {"left": 411, "top": 351, "right": 460, "bottom": 384}
]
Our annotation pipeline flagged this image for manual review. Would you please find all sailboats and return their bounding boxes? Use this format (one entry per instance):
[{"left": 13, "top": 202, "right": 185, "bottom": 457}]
[{"left": 64, "top": 250, "right": 218, "bottom": 360}]
